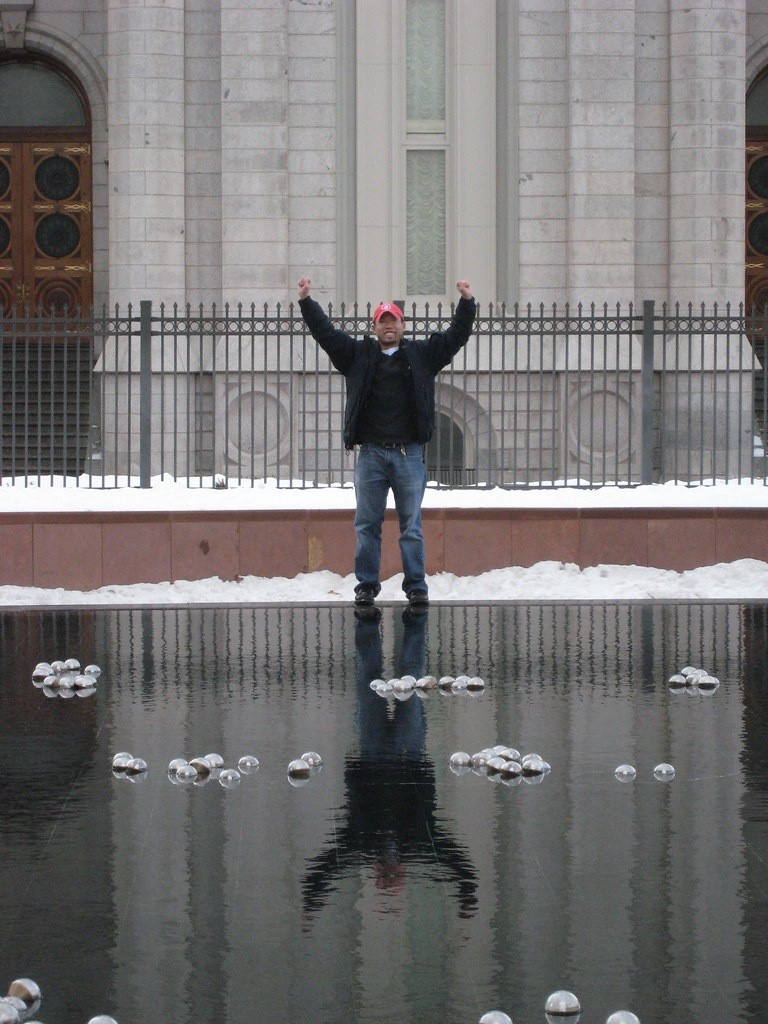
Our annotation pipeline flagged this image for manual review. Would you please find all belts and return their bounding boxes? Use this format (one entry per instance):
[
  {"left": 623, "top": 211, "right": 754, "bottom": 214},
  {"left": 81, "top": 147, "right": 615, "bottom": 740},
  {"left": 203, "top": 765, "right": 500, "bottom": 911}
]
[{"left": 372, "top": 441, "right": 408, "bottom": 448}]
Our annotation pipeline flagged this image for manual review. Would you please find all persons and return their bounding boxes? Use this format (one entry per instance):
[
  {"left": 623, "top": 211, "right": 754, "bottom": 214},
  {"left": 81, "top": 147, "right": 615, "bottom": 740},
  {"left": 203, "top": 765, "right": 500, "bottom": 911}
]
[
  {"left": 298, "top": 606, "right": 480, "bottom": 936},
  {"left": 297, "top": 276, "right": 476, "bottom": 605}
]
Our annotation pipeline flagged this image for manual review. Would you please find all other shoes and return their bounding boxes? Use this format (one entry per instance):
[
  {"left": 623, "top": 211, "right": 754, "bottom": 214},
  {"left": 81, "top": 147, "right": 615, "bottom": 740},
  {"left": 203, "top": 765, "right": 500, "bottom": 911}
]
[
  {"left": 353, "top": 606, "right": 381, "bottom": 620},
  {"left": 354, "top": 588, "right": 374, "bottom": 605},
  {"left": 404, "top": 604, "right": 429, "bottom": 615},
  {"left": 409, "top": 590, "right": 428, "bottom": 604}
]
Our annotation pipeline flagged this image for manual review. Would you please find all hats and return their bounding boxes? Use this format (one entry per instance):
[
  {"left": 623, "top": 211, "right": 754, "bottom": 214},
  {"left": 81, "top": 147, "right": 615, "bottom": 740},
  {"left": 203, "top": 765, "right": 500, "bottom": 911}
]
[{"left": 373, "top": 303, "right": 403, "bottom": 324}]
